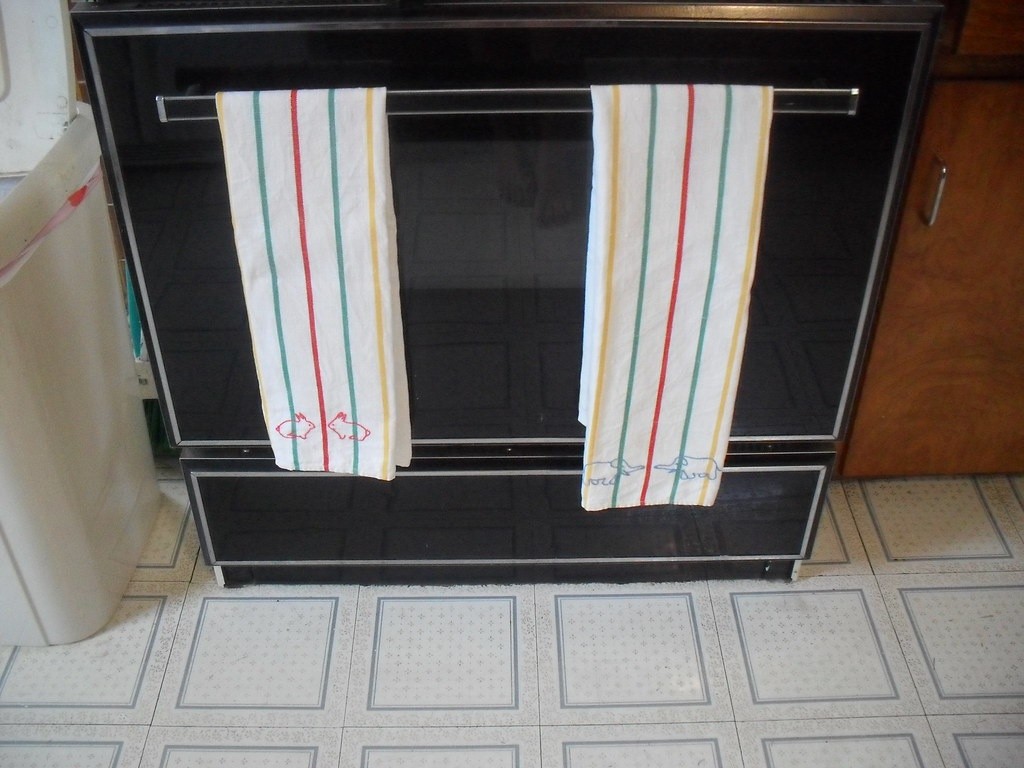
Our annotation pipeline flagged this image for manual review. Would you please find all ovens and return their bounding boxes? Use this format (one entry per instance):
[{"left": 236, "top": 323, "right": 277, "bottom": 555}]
[{"left": 69, "top": 9, "right": 938, "bottom": 587}]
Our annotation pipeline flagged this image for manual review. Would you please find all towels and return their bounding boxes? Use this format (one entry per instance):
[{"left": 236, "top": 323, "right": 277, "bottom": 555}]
[
  {"left": 576, "top": 85, "right": 775, "bottom": 515},
  {"left": 213, "top": 86, "right": 415, "bottom": 483}
]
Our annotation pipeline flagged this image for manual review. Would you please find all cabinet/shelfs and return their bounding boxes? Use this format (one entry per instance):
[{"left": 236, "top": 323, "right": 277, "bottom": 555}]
[{"left": 829, "top": 0, "right": 1024, "bottom": 482}]
